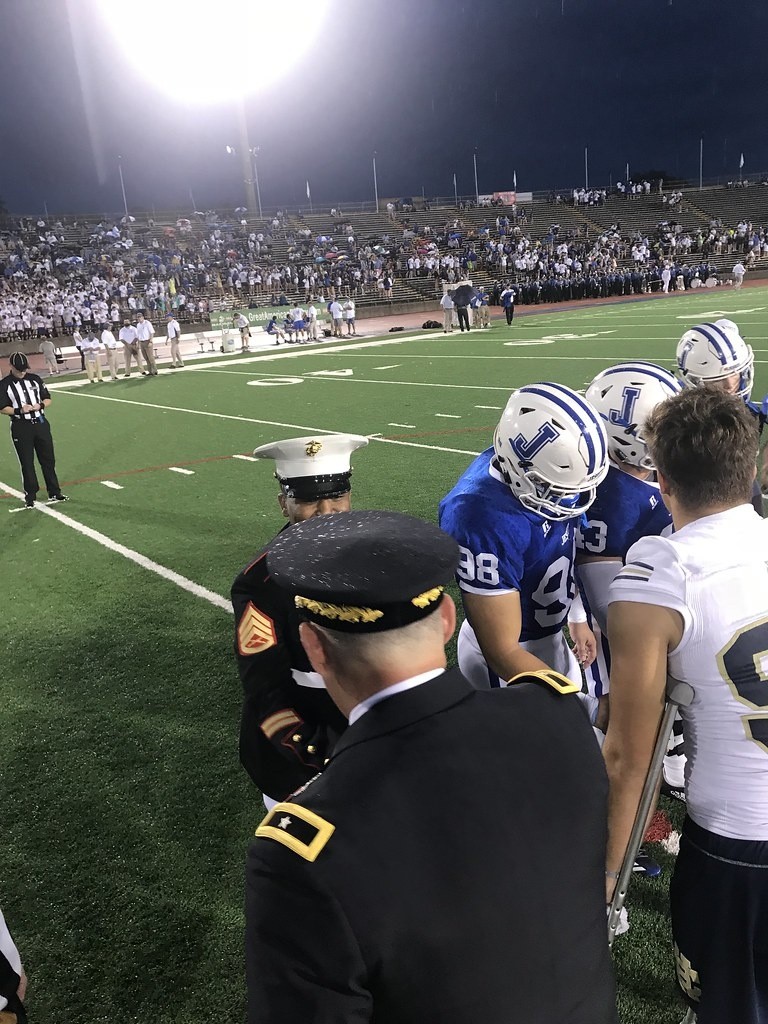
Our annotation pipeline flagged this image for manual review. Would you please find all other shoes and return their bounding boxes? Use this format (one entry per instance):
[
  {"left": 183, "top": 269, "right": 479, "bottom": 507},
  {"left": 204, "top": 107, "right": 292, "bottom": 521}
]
[
  {"left": 48, "top": 494, "right": 69, "bottom": 501},
  {"left": 25, "top": 501, "right": 34, "bottom": 508},
  {"left": 169, "top": 364, "right": 176, "bottom": 369},
  {"left": 91, "top": 372, "right": 158, "bottom": 383},
  {"left": 276, "top": 332, "right": 358, "bottom": 345},
  {"left": 179, "top": 365, "right": 184, "bottom": 368}
]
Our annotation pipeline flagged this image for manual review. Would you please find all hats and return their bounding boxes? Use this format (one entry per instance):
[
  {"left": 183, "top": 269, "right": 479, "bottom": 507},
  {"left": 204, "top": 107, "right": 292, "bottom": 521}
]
[
  {"left": 137, "top": 313, "right": 143, "bottom": 317},
  {"left": 10, "top": 352, "right": 31, "bottom": 371},
  {"left": 103, "top": 322, "right": 113, "bottom": 328},
  {"left": 253, "top": 434, "right": 369, "bottom": 500},
  {"left": 165, "top": 312, "right": 175, "bottom": 316},
  {"left": 266, "top": 510, "right": 460, "bottom": 634},
  {"left": 122, "top": 319, "right": 132, "bottom": 326}
]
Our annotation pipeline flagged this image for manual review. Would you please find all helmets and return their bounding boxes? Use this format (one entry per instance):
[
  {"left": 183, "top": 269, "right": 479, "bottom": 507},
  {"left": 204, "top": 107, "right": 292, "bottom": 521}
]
[
  {"left": 677, "top": 323, "right": 754, "bottom": 403},
  {"left": 492, "top": 381, "right": 610, "bottom": 521},
  {"left": 585, "top": 361, "right": 683, "bottom": 470}
]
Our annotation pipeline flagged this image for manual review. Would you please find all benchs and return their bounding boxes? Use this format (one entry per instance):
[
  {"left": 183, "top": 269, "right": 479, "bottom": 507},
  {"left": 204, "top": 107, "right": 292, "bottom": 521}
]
[{"left": 0, "top": 181, "right": 768, "bottom": 341}]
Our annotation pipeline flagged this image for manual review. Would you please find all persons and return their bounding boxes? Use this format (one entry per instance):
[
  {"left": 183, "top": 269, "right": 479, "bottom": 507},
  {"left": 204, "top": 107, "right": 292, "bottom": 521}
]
[
  {"left": 0, "top": 352, "right": 70, "bottom": 510},
  {"left": 231, "top": 319, "right": 768, "bottom": 1023},
  {"left": 0, "top": 909, "right": 28, "bottom": 1024},
  {"left": 0, "top": 179, "right": 768, "bottom": 383}
]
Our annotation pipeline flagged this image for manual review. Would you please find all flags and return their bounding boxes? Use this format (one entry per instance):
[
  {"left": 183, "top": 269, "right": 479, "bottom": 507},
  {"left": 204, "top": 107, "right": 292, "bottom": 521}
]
[{"left": 739, "top": 153, "right": 744, "bottom": 168}]
